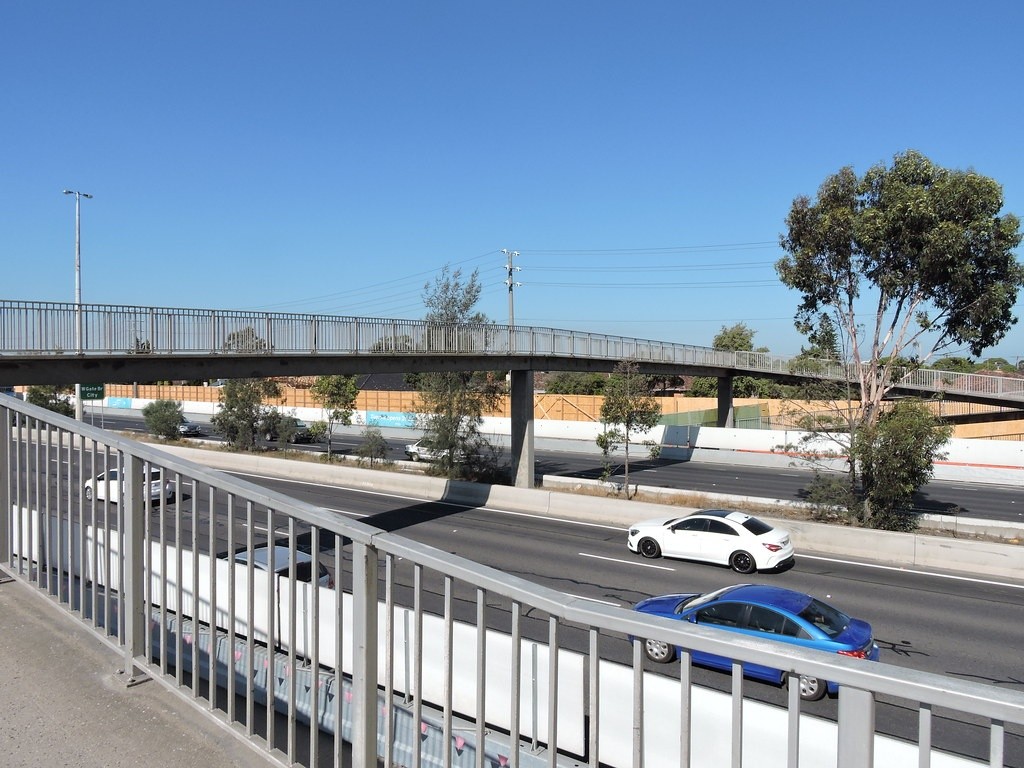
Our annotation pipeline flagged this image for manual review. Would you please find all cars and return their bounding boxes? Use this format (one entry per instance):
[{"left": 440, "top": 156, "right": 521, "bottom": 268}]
[
  {"left": 176, "top": 416, "right": 201, "bottom": 438},
  {"left": 628, "top": 584, "right": 880, "bottom": 700},
  {"left": 222, "top": 545, "right": 334, "bottom": 591},
  {"left": 86, "top": 466, "right": 173, "bottom": 509},
  {"left": 627, "top": 509, "right": 794, "bottom": 573},
  {"left": 405, "top": 438, "right": 485, "bottom": 468}
]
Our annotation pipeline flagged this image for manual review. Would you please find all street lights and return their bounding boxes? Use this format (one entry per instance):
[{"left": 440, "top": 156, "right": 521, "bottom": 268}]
[{"left": 64, "top": 191, "right": 93, "bottom": 354}]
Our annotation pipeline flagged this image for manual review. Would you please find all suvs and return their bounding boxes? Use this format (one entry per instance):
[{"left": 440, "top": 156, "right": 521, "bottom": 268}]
[{"left": 266, "top": 419, "right": 313, "bottom": 443}]
